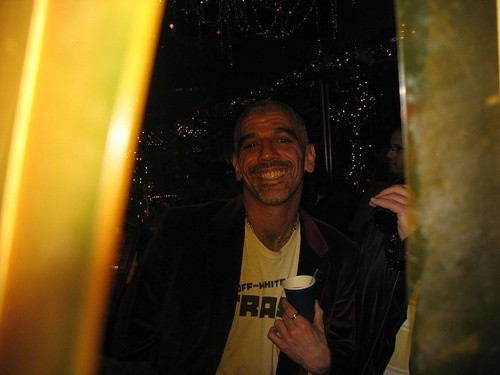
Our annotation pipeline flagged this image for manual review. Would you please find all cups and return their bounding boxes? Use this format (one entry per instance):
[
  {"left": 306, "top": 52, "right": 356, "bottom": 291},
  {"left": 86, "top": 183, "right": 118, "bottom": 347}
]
[{"left": 281, "top": 275, "right": 317, "bottom": 324}]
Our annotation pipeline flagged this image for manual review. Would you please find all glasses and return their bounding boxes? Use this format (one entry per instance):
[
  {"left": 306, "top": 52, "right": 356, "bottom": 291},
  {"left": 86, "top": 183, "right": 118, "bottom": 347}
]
[{"left": 388, "top": 144, "right": 402, "bottom": 154}]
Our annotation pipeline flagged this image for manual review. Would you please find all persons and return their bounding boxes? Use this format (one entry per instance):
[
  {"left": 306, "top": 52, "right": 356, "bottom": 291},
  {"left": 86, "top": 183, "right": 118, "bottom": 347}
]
[
  {"left": 368, "top": 185, "right": 420, "bottom": 375},
  {"left": 112, "top": 100, "right": 362, "bottom": 375},
  {"left": 357, "top": 127, "right": 404, "bottom": 273}
]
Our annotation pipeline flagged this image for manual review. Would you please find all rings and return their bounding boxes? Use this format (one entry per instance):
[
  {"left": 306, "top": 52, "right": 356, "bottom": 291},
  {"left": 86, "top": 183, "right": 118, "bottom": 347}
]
[{"left": 291, "top": 312, "right": 300, "bottom": 319}]
